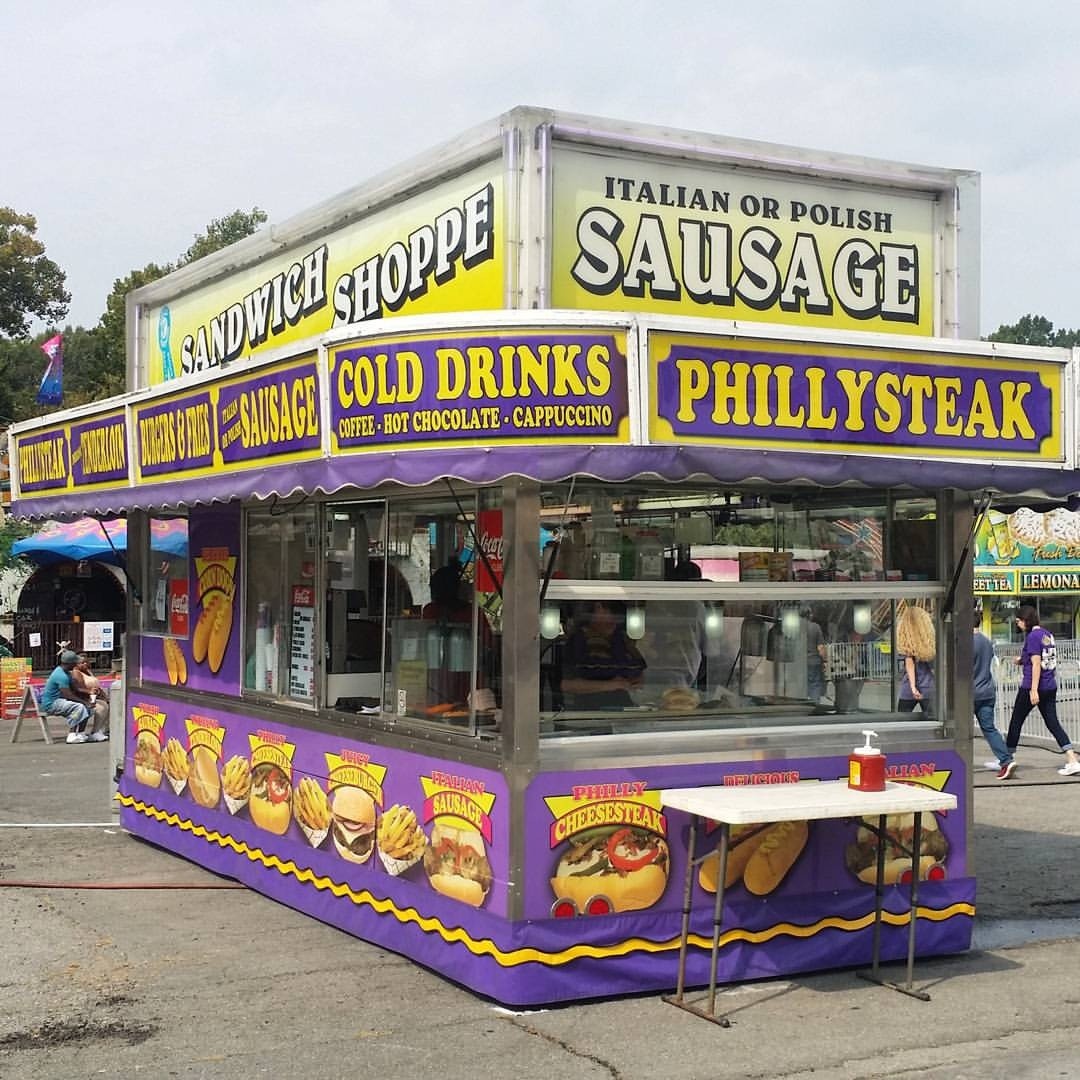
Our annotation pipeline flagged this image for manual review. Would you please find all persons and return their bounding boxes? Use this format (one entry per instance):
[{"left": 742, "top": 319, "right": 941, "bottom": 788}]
[
  {"left": 974, "top": 610, "right": 1019, "bottom": 779},
  {"left": 558, "top": 600, "right": 648, "bottom": 711},
  {"left": 983, "top": 607, "right": 1080, "bottom": 776},
  {"left": 896, "top": 606, "right": 937, "bottom": 713},
  {"left": 422, "top": 566, "right": 495, "bottom": 704},
  {"left": 72, "top": 653, "right": 110, "bottom": 741},
  {"left": 40, "top": 651, "right": 96, "bottom": 743},
  {"left": 801, "top": 606, "right": 828, "bottom": 700},
  {"left": 644, "top": 563, "right": 708, "bottom": 704}
]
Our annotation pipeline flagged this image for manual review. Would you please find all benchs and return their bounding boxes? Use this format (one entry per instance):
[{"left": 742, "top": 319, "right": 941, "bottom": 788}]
[{"left": 5, "top": 686, "right": 61, "bottom": 744}]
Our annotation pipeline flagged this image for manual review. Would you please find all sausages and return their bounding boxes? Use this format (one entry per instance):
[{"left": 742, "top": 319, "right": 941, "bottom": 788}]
[{"left": 424, "top": 702, "right": 459, "bottom": 714}]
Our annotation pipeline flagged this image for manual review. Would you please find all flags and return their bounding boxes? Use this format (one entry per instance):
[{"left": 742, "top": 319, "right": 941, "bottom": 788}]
[{"left": 35, "top": 333, "right": 63, "bottom": 408}]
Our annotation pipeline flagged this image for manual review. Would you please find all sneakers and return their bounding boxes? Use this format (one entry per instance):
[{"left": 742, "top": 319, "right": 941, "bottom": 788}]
[
  {"left": 66, "top": 733, "right": 88, "bottom": 743},
  {"left": 984, "top": 758, "right": 1001, "bottom": 771},
  {"left": 995, "top": 761, "right": 1019, "bottom": 780},
  {"left": 1057, "top": 762, "right": 1080, "bottom": 776},
  {"left": 88, "top": 732, "right": 105, "bottom": 742}
]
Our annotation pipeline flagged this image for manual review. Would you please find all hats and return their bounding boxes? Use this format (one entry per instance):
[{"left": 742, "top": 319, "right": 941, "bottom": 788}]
[{"left": 61, "top": 650, "right": 81, "bottom": 664}]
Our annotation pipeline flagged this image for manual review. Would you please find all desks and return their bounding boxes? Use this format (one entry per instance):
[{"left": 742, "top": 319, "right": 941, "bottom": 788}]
[{"left": 659, "top": 780, "right": 960, "bottom": 1028}]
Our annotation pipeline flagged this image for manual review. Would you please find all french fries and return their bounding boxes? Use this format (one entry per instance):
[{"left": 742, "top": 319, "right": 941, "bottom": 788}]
[
  {"left": 221, "top": 754, "right": 252, "bottom": 800},
  {"left": 376, "top": 804, "right": 425, "bottom": 860},
  {"left": 293, "top": 777, "right": 330, "bottom": 830},
  {"left": 162, "top": 736, "right": 189, "bottom": 780}
]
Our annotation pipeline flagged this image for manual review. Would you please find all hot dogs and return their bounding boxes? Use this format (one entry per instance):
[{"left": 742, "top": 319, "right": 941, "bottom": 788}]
[
  {"left": 192, "top": 594, "right": 233, "bottom": 672},
  {"left": 663, "top": 687, "right": 704, "bottom": 711},
  {"left": 699, "top": 820, "right": 810, "bottom": 892},
  {"left": 163, "top": 639, "right": 187, "bottom": 685}
]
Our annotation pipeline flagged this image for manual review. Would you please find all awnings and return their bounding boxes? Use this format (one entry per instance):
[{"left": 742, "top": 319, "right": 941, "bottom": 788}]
[
  {"left": 5, "top": 310, "right": 1080, "bottom": 529},
  {"left": 12, "top": 517, "right": 190, "bottom": 571}
]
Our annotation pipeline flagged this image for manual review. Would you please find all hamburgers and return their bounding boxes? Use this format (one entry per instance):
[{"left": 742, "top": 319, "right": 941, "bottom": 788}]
[
  {"left": 423, "top": 824, "right": 492, "bottom": 907},
  {"left": 549, "top": 833, "right": 670, "bottom": 911},
  {"left": 189, "top": 747, "right": 221, "bottom": 809},
  {"left": 332, "top": 788, "right": 376, "bottom": 863},
  {"left": 133, "top": 731, "right": 163, "bottom": 789},
  {"left": 846, "top": 810, "right": 947, "bottom": 885},
  {"left": 249, "top": 763, "right": 292, "bottom": 835}
]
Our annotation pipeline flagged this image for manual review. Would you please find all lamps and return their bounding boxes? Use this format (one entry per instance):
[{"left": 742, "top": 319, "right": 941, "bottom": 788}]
[
  {"left": 627, "top": 598, "right": 646, "bottom": 639},
  {"left": 540, "top": 598, "right": 562, "bottom": 640},
  {"left": 780, "top": 599, "right": 802, "bottom": 638},
  {"left": 852, "top": 599, "right": 871, "bottom": 634},
  {"left": 704, "top": 599, "right": 724, "bottom": 636}
]
